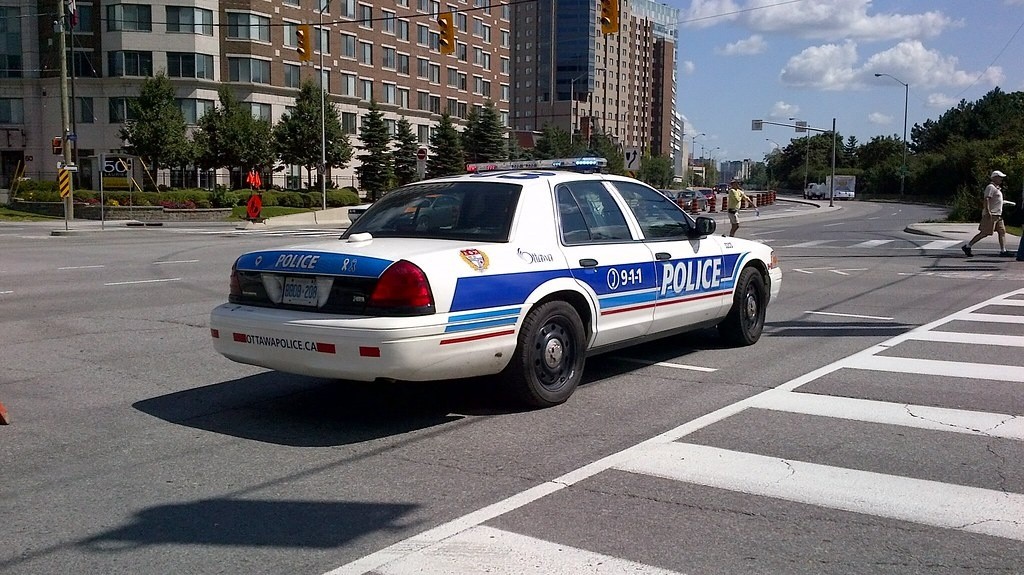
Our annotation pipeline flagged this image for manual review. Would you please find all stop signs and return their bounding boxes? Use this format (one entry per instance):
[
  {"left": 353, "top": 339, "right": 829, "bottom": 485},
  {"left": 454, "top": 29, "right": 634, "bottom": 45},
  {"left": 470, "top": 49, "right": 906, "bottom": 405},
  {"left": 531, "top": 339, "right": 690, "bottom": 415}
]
[{"left": 415, "top": 149, "right": 426, "bottom": 160}]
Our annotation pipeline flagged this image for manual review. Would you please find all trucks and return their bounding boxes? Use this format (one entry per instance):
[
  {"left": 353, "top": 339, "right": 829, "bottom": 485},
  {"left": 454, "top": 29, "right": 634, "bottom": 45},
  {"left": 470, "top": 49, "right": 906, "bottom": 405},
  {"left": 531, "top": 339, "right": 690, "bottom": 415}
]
[{"left": 825, "top": 176, "right": 857, "bottom": 201}]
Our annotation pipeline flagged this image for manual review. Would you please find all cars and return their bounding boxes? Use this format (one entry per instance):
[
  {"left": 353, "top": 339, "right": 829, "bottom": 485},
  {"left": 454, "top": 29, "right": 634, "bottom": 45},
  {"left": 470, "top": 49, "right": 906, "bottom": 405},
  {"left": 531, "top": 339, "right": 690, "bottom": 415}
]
[
  {"left": 714, "top": 183, "right": 730, "bottom": 193},
  {"left": 656, "top": 186, "right": 718, "bottom": 211},
  {"left": 348, "top": 194, "right": 462, "bottom": 234},
  {"left": 211, "top": 158, "right": 782, "bottom": 406}
]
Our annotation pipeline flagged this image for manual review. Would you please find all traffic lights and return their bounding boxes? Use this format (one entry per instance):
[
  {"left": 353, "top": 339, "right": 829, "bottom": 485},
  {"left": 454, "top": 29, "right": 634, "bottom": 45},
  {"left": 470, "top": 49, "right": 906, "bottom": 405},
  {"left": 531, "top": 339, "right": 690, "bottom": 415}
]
[
  {"left": 600, "top": 0, "right": 619, "bottom": 33},
  {"left": 52, "top": 139, "right": 64, "bottom": 154},
  {"left": 296, "top": 25, "right": 310, "bottom": 61}
]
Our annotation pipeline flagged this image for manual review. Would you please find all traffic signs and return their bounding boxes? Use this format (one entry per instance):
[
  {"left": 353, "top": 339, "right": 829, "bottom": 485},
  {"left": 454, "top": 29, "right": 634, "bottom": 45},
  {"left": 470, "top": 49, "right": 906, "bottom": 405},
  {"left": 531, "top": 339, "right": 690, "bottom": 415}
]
[{"left": 61, "top": 165, "right": 78, "bottom": 173}]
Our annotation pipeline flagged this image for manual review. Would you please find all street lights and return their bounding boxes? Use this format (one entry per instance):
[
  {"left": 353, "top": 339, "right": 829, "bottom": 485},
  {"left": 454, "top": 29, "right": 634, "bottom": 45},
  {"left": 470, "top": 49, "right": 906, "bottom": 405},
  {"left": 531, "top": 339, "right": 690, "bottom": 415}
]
[
  {"left": 680, "top": 133, "right": 721, "bottom": 187},
  {"left": 766, "top": 138, "right": 779, "bottom": 148},
  {"left": 874, "top": 73, "right": 909, "bottom": 199},
  {"left": 570, "top": 66, "right": 607, "bottom": 149},
  {"left": 788, "top": 117, "right": 810, "bottom": 194},
  {"left": 438, "top": 13, "right": 455, "bottom": 54}
]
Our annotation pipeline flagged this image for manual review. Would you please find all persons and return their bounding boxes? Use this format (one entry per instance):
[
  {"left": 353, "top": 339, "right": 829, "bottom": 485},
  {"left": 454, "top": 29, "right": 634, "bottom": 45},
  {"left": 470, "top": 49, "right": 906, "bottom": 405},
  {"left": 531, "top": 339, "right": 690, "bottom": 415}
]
[
  {"left": 961, "top": 171, "right": 1015, "bottom": 257},
  {"left": 728, "top": 180, "right": 757, "bottom": 237}
]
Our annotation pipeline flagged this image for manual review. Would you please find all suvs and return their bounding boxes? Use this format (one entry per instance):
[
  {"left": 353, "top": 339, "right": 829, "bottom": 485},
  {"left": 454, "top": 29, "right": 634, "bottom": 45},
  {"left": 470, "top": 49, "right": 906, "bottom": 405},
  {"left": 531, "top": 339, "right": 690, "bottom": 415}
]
[{"left": 803, "top": 183, "right": 823, "bottom": 199}]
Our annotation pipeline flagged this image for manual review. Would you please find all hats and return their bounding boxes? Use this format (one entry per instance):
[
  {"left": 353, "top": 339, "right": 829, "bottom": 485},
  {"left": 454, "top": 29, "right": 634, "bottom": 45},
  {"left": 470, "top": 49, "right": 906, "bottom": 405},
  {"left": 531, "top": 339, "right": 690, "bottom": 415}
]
[{"left": 992, "top": 170, "right": 1007, "bottom": 177}]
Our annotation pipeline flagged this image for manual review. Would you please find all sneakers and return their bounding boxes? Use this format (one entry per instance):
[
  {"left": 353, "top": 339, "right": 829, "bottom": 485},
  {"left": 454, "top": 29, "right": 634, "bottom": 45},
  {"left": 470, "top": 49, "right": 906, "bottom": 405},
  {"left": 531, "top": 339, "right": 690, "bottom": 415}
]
[
  {"left": 962, "top": 244, "right": 973, "bottom": 256},
  {"left": 999, "top": 251, "right": 1015, "bottom": 256}
]
